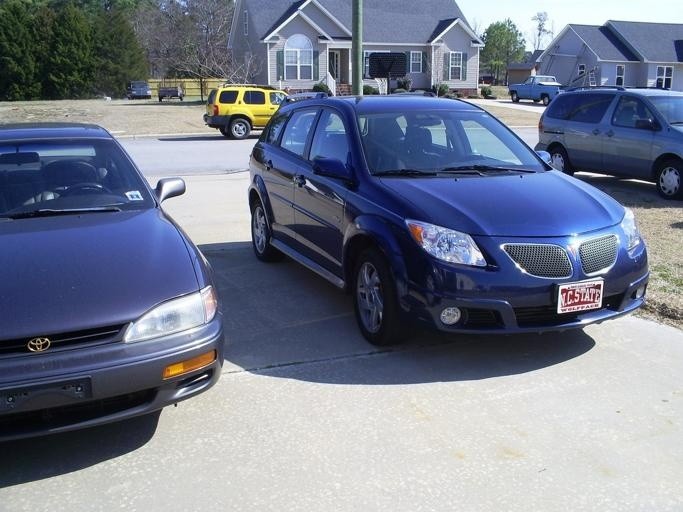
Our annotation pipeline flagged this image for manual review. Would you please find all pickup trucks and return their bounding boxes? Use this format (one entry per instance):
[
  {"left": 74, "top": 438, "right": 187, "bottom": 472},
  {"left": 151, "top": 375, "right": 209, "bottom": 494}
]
[{"left": 508, "top": 75, "right": 578, "bottom": 107}]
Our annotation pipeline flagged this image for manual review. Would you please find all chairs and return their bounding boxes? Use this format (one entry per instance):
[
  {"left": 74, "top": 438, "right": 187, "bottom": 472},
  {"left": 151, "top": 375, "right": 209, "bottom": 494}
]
[
  {"left": 284, "top": 115, "right": 445, "bottom": 172},
  {"left": 615, "top": 106, "right": 638, "bottom": 127},
  {"left": 40, "top": 158, "right": 103, "bottom": 204}
]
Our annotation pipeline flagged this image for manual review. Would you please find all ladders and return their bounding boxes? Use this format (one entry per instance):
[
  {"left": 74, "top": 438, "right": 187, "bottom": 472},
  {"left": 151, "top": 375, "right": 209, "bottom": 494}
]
[{"left": 583, "top": 68, "right": 597, "bottom": 88}]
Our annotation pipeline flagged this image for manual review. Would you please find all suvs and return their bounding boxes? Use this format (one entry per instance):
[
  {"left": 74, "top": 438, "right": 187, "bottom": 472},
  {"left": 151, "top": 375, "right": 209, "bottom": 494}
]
[
  {"left": 202, "top": 83, "right": 333, "bottom": 140},
  {"left": 248, "top": 92, "right": 653, "bottom": 346},
  {"left": 533, "top": 84, "right": 682, "bottom": 199},
  {"left": 125, "top": 80, "right": 151, "bottom": 100}
]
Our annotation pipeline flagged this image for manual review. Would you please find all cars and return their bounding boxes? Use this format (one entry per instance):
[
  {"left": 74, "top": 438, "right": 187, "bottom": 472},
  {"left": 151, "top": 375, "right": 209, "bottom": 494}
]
[{"left": 0, "top": 121, "right": 225, "bottom": 443}]
